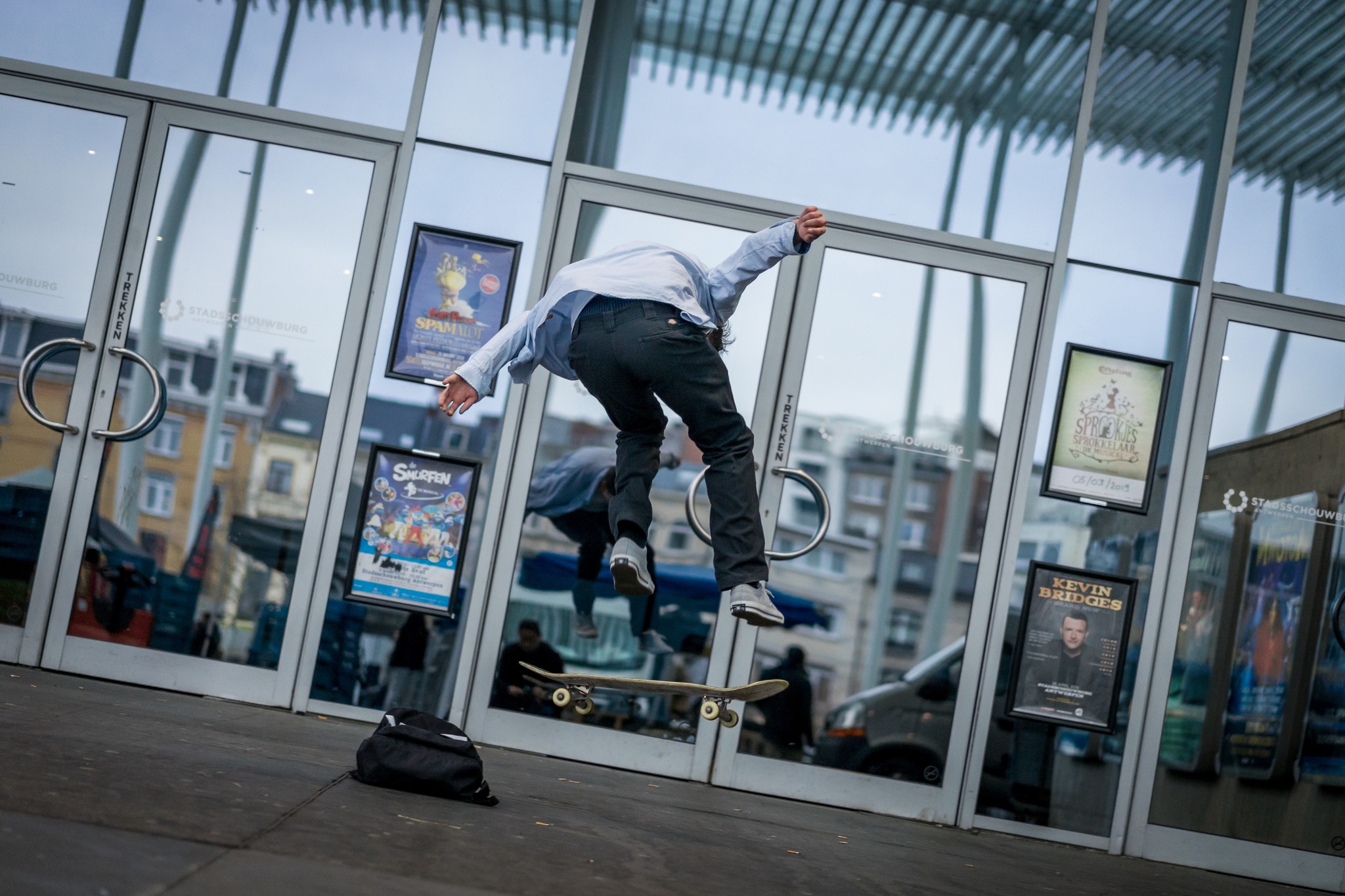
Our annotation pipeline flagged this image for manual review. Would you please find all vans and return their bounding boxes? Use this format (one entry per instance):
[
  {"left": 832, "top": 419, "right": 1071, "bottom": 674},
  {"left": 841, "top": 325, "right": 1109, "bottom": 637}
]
[{"left": 819, "top": 634, "right": 1054, "bottom": 823}]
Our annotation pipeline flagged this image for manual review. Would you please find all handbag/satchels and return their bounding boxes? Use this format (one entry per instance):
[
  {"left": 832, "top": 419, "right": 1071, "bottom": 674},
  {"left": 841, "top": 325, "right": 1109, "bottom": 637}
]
[{"left": 352, "top": 708, "right": 499, "bottom": 806}]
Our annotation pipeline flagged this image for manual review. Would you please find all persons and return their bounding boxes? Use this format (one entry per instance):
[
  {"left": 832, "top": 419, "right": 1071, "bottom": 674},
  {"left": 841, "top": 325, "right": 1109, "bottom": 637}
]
[
  {"left": 438, "top": 205, "right": 826, "bottom": 627},
  {"left": 487, "top": 618, "right": 564, "bottom": 719},
  {"left": 379, "top": 611, "right": 429, "bottom": 714},
  {"left": 523, "top": 446, "right": 681, "bottom": 655},
  {"left": 750, "top": 645, "right": 815, "bottom": 763},
  {"left": 1021, "top": 608, "right": 1107, "bottom": 719}
]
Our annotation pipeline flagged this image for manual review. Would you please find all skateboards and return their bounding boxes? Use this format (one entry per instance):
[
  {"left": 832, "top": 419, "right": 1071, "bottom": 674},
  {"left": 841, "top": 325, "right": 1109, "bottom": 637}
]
[{"left": 519, "top": 662, "right": 789, "bottom": 723}]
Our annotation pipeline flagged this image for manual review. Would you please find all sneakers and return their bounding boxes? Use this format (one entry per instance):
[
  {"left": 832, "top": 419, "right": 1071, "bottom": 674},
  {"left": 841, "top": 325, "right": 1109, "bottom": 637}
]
[
  {"left": 730, "top": 581, "right": 786, "bottom": 626},
  {"left": 635, "top": 631, "right": 673, "bottom": 655},
  {"left": 569, "top": 611, "right": 598, "bottom": 639},
  {"left": 611, "top": 538, "right": 656, "bottom": 596}
]
